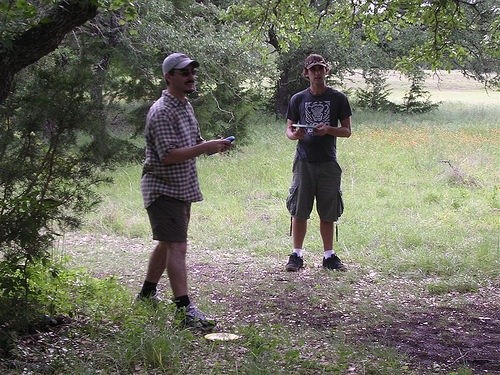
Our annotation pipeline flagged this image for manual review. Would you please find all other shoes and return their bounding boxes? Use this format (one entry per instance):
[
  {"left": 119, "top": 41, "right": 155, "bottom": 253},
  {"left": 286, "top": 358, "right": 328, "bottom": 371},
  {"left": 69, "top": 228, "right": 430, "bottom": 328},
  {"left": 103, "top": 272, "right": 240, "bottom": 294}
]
[
  {"left": 176, "top": 306, "right": 217, "bottom": 331},
  {"left": 286, "top": 252, "right": 303, "bottom": 271},
  {"left": 322, "top": 254, "right": 347, "bottom": 270}
]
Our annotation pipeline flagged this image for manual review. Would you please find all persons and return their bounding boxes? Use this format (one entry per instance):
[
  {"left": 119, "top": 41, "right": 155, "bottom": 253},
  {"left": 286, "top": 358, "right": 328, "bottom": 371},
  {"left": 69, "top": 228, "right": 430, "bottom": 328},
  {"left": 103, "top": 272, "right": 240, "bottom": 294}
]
[
  {"left": 286, "top": 54, "right": 352, "bottom": 270},
  {"left": 131, "top": 52, "right": 232, "bottom": 332}
]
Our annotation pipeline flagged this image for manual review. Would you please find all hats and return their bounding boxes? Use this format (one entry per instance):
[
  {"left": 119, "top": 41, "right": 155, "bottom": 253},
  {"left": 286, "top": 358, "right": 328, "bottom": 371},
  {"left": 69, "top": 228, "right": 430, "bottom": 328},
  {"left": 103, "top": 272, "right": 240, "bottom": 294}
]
[
  {"left": 304, "top": 53, "right": 327, "bottom": 69},
  {"left": 162, "top": 53, "right": 200, "bottom": 73}
]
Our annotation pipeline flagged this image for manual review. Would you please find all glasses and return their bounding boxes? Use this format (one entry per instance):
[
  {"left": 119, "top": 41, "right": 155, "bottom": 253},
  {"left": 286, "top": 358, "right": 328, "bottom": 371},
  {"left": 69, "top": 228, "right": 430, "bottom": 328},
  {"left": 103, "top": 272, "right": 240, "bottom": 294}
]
[{"left": 170, "top": 70, "right": 196, "bottom": 76}]
